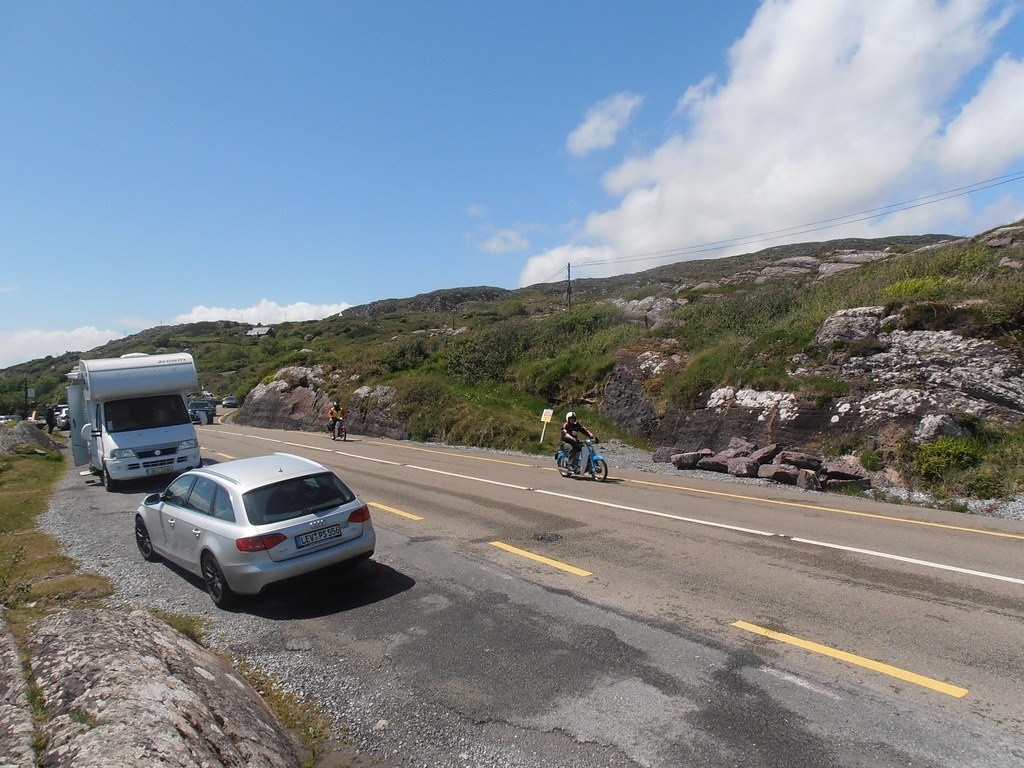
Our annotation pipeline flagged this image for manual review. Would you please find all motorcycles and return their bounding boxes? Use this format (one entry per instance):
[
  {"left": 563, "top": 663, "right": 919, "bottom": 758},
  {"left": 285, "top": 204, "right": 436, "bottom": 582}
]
[{"left": 554, "top": 439, "right": 608, "bottom": 482}]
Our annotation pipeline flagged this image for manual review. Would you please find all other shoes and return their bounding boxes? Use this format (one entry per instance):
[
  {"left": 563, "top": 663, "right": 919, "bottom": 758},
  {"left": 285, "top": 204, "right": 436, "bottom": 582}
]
[
  {"left": 331, "top": 433, "right": 335, "bottom": 439},
  {"left": 569, "top": 464, "right": 575, "bottom": 471}
]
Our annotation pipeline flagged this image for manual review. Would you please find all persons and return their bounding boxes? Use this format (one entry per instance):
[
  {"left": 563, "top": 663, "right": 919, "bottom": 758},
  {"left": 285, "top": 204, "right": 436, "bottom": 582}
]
[
  {"left": 327, "top": 401, "right": 348, "bottom": 440},
  {"left": 45, "top": 404, "right": 58, "bottom": 435},
  {"left": 559, "top": 412, "right": 599, "bottom": 471}
]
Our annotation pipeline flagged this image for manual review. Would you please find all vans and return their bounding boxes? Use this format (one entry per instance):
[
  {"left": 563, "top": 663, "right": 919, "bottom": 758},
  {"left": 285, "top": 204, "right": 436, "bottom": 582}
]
[
  {"left": 0, "top": 415, "right": 23, "bottom": 425},
  {"left": 64, "top": 352, "right": 203, "bottom": 493}
]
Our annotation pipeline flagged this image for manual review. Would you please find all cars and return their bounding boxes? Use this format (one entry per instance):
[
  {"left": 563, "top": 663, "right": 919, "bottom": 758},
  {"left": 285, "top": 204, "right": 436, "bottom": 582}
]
[
  {"left": 55, "top": 408, "right": 70, "bottom": 431},
  {"left": 222, "top": 395, "right": 238, "bottom": 408},
  {"left": 187, "top": 401, "right": 215, "bottom": 424},
  {"left": 54, "top": 403, "right": 68, "bottom": 426},
  {"left": 200, "top": 398, "right": 216, "bottom": 415},
  {"left": 134, "top": 451, "right": 377, "bottom": 605}
]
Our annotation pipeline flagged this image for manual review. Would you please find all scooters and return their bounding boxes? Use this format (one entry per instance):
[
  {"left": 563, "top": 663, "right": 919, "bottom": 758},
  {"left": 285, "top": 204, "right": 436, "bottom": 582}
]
[{"left": 326, "top": 410, "right": 350, "bottom": 442}]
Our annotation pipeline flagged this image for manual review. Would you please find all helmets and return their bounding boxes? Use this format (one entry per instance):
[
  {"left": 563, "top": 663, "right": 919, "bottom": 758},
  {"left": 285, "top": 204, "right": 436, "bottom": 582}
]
[
  {"left": 333, "top": 402, "right": 339, "bottom": 408},
  {"left": 566, "top": 412, "right": 577, "bottom": 424}
]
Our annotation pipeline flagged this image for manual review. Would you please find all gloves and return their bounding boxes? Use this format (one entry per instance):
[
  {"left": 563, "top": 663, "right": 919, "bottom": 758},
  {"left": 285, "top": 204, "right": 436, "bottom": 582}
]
[
  {"left": 591, "top": 436, "right": 599, "bottom": 444},
  {"left": 575, "top": 440, "right": 582, "bottom": 449}
]
